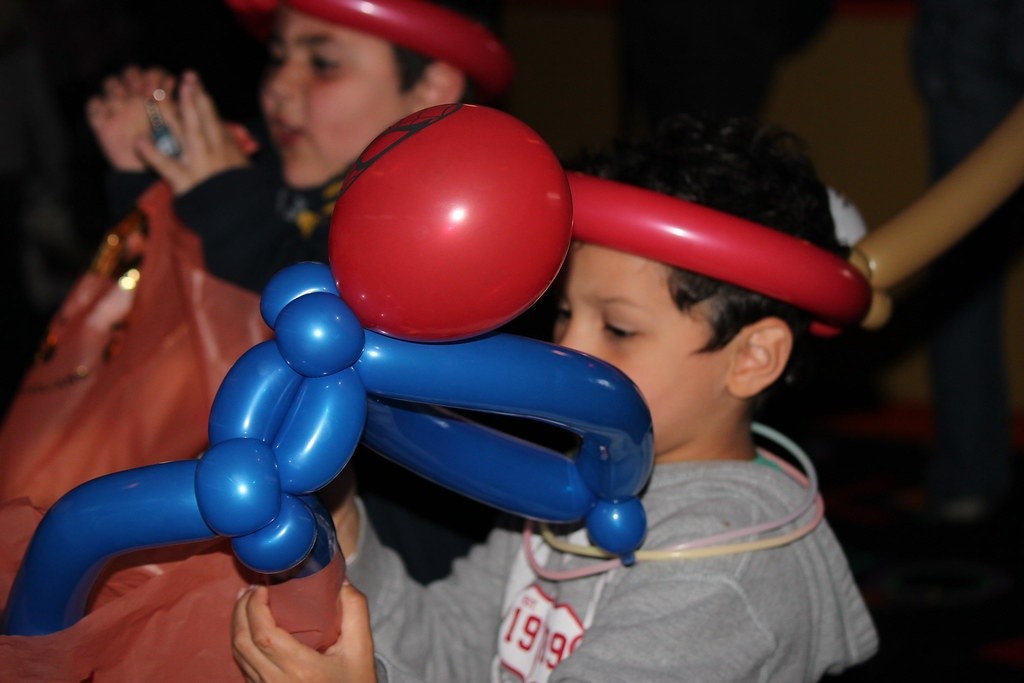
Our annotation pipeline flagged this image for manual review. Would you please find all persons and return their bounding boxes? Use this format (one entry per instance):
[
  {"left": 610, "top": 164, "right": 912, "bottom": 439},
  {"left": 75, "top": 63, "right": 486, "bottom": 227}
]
[
  {"left": 608, "top": 1, "right": 840, "bottom": 142},
  {"left": 228, "top": 168, "right": 881, "bottom": 682},
  {"left": 903, "top": 0, "right": 1024, "bottom": 530},
  {"left": 82, "top": 0, "right": 584, "bottom": 584}
]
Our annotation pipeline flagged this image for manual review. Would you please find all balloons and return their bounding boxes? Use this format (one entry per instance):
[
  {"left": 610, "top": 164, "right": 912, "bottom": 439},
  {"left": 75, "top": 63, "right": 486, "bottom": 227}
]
[
  {"left": 566, "top": 101, "right": 1024, "bottom": 334},
  {"left": 0, "top": 100, "right": 655, "bottom": 634},
  {"left": 230, "top": 0, "right": 516, "bottom": 104}
]
